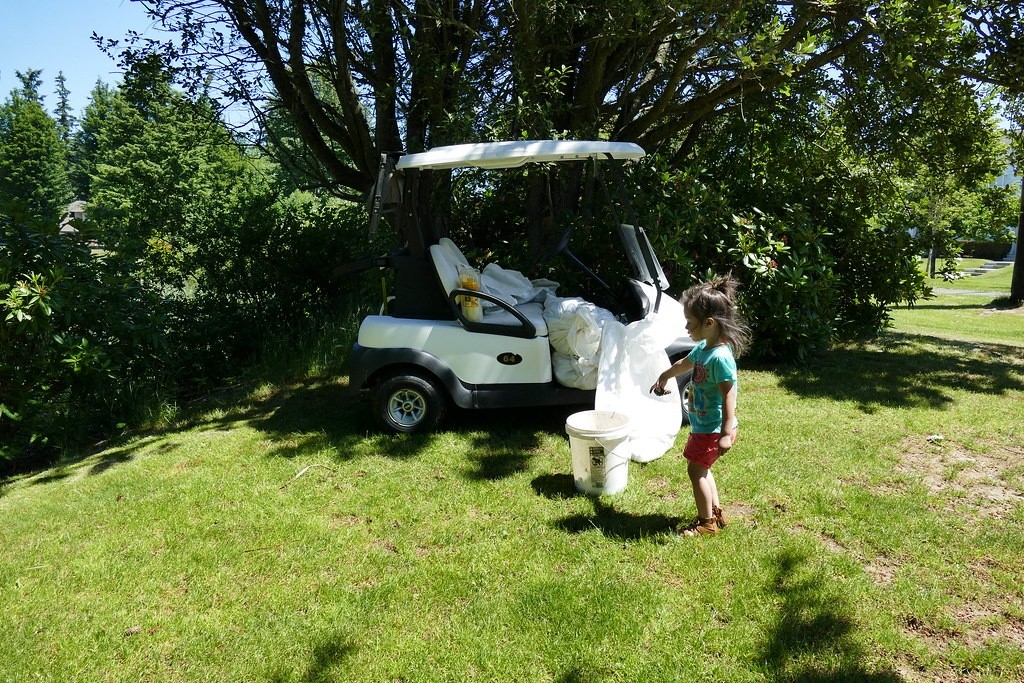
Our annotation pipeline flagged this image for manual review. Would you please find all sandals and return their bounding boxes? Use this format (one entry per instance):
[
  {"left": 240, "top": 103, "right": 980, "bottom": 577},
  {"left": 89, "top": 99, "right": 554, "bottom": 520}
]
[
  {"left": 712, "top": 505, "right": 727, "bottom": 528},
  {"left": 679, "top": 516, "right": 717, "bottom": 538}
]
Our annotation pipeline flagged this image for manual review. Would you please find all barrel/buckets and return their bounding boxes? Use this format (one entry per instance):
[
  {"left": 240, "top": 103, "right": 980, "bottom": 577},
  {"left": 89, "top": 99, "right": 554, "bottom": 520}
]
[{"left": 565, "top": 410, "right": 632, "bottom": 496}]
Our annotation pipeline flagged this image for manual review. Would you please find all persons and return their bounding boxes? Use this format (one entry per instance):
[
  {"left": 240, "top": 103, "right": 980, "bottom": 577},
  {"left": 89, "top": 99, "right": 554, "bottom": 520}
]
[{"left": 650, "top": 278, "right": 744, "bottom": 537}]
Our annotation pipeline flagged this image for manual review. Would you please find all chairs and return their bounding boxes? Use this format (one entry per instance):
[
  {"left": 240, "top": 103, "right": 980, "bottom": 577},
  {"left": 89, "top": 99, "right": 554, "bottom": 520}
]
[
  {"left": 430, "top": 243, "right": 550, "bottom": 338},
  {"left": 440, "top": 237, "right": 543, "bottom": 308}
]
